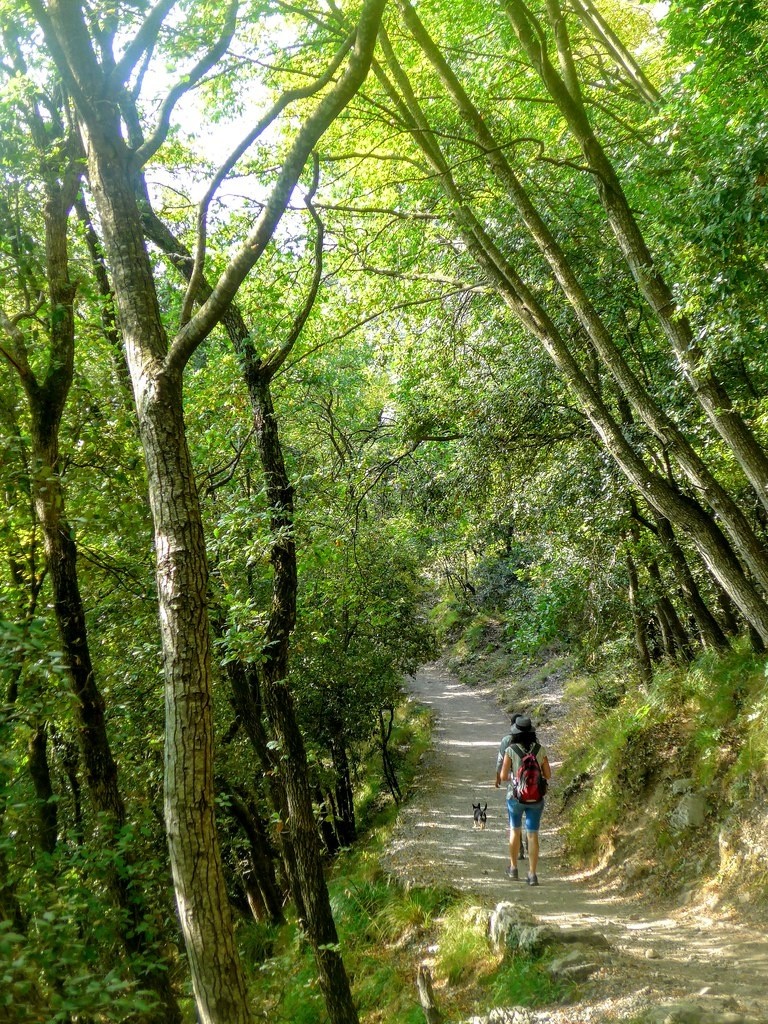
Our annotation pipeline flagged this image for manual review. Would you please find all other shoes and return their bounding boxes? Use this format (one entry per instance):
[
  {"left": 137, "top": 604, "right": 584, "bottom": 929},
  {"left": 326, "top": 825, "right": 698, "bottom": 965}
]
[{"left": 518, "top": 854, "right": 525, "bottom": 860}]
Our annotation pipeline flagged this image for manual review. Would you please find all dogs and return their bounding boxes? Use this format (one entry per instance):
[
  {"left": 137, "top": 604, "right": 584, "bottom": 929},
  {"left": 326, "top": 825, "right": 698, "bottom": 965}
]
[
  {"left": 556, "top": 771, "right": 590, "bottom": 816},
  {"left": 471, "top": 801, "right": 489, "bottom": 829}
]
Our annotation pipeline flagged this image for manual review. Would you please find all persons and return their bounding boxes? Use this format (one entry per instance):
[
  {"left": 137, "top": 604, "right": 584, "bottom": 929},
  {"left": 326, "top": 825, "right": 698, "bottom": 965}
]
[
  {"left": 499, "top": 716, "right": 550, "bottom": 885},
  {"left": 496, "top": 714, "right": 542, "bottom": 860}
]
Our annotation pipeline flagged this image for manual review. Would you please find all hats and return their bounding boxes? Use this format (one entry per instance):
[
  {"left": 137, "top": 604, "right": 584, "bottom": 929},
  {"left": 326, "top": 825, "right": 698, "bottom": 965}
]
[{"left": 511, "top": 716, "right": 537, "bottom": 735}]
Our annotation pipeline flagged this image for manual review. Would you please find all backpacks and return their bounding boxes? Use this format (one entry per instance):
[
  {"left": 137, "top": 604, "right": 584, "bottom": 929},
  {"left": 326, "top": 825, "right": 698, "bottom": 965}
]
[{"left": 509, "top": 743, "right": 548, "bottom": 805}]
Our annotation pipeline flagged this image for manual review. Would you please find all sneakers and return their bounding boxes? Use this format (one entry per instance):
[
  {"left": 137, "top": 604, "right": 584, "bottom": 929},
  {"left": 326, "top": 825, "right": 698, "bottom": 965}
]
[
  {"left": 525, "top": 873, "right": 540, "bottom": 887},
  {"left": 505, "top": 866, "right": 518, "bottom": 880}
]
